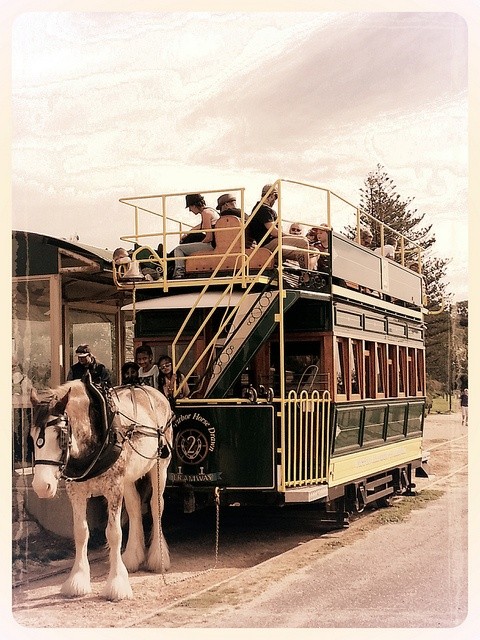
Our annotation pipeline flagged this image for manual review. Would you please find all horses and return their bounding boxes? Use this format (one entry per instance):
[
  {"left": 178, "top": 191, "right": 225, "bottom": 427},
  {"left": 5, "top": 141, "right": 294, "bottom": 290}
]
[{"left": 27, "top": 379, "right": 174, "bottom": 603}]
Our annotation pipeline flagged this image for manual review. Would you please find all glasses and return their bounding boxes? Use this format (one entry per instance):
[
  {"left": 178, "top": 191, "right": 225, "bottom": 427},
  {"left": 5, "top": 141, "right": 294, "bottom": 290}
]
[
  {"left": 159, "top": 363, "right": 172, "bottom": 369},
  {"left": 292, "top": 228, "right": 301, "bottom": 232}
]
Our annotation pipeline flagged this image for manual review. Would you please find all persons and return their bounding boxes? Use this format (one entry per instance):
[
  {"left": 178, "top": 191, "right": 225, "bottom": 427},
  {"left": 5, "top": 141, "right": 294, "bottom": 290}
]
[
  {"left": 249, "top": 184, "right": 320, "bottom": 286},
  {"left": 121, "top": 362, "right": 140, "bottom": 386},
  {"left": 306, "top": 229, "right": 316, "bottom": 243},
  {"left": 14, "top": 373, "right": 38, "bottom": 394},
  {"left": 215, "top": 193, "right": 248, "bottom": 222},
  {"left": 310, "top": 223, "right": 333, "bottom": 252},
  {"left": 173, "top": 194, "right": 219, "bottom": 278},
  {"left": 67, "top": 343, "right": 114, "bottom": 387},
  {"left": 374, "top": 237, "right": 397, "bottom": 260},
  {"left": 134, "top": 344, "right": 160, "bottom": 391},
  {"left": 458, "top": 389, "right": 468, "bottom": 426},
  {"left": 289, "top": 223, "right": 302, "bottom": 235},
  {"left": 406, "top": 260, "right": 418, "bottom": 271},
  {"left": 156, "top": 355, "right": 190, "bottom": 400},
  {"left": 353, "top": 229, "right": 373, "bottom": 246}
]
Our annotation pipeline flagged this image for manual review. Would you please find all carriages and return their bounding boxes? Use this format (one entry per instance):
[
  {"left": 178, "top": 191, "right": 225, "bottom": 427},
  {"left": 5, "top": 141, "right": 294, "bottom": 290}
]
[{"left": 30, "top": 179, "right": 430, "bottom": 601}]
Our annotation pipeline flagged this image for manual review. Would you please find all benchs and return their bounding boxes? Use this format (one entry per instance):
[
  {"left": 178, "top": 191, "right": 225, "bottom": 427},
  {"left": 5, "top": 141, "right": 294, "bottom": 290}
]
[{"left": 184, "top": 216, "right": 273, "bottom": 271}]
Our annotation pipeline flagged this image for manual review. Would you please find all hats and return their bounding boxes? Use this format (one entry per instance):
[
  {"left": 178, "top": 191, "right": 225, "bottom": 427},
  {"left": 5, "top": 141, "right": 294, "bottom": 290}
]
[
  {"left": 312, "top": 223, "right": 333, "bottom": 232},
  {"left": 262, "top": 184, "right": 278, "bottom": 195},
  {"left": 185, "top": 194, "right": 204, "bottom": 208},
  {"left": 216, "top": 194, "right": 236, "bottom": 210},
  {"left": 76, "top": 344, "right": 90, "bottom": 357},
  {"left": 12, "top": 372, "right": 24, "bottom": 384}
]
